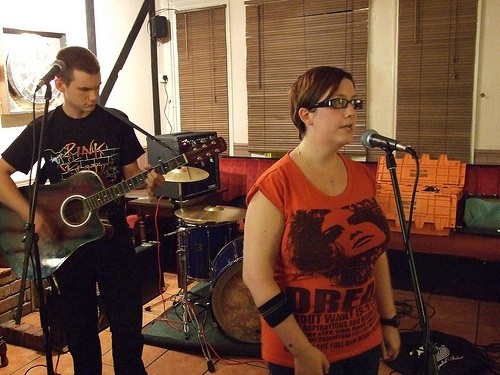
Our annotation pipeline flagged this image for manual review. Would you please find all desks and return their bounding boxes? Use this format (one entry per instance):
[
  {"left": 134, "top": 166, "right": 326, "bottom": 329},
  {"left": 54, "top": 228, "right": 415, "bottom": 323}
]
[{"left": 123, "top": 185, "right": 230, "bottom": 342}]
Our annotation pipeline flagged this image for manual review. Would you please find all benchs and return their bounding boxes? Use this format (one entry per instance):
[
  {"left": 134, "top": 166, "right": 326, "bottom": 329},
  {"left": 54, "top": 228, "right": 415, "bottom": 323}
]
[{"left": 129, "top": 155, "right": 500, "bottom": 287}]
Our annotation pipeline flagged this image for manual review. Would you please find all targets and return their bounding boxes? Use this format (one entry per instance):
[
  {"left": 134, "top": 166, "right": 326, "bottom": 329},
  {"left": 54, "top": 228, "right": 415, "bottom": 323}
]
[{"left": 5, "top": 33, "right": 60, "bottom": 103}]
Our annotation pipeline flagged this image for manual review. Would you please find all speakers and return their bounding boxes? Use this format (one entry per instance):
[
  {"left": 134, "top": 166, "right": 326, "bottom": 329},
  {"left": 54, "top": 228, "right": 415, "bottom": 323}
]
[{"left": 151, "top": 15, "right": 167, "bottom": 38}]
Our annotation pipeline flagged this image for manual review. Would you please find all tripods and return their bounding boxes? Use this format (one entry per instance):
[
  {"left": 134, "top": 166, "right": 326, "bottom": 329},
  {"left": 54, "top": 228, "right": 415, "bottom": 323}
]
[{"left": 149, "top": 182, "right": 207, "bottom": 338}]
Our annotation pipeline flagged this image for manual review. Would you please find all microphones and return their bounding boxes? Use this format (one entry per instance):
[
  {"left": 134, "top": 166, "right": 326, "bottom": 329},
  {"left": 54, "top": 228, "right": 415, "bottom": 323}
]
[
  {"left": 36, "top": 59, "right": 66, "bottom": 91},
  {"left": 361, "top": 128, "right": 416, "bottom": 155}
]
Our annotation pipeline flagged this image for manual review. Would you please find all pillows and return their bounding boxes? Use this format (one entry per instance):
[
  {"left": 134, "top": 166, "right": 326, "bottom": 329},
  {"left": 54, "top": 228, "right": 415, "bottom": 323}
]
[{"left": 457, "top": 192, "right": 500, "bottom": 236}]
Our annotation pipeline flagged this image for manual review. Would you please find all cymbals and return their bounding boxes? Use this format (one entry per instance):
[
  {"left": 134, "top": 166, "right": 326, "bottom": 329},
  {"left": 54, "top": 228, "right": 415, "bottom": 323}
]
[
  {"left": 174, "top": 205, "right": 247, "bottom": 223},
  {"left": 162, "top": 165, "right": 210, "bottom": 183}
]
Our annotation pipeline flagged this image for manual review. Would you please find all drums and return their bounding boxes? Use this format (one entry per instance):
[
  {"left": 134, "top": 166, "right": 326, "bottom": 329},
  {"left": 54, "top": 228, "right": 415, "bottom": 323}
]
[
  {"left": 182, "top": 221, "right": 239, "bottom": 281},
  {"left": 206, "top": 236, "right": 262, "bottom": 345}
]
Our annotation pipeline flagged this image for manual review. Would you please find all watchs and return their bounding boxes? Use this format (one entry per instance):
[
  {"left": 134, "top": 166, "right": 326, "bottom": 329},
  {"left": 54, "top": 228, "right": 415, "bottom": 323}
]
[{"left": 381, "top": 315, "right": 400, "bottom": 327}]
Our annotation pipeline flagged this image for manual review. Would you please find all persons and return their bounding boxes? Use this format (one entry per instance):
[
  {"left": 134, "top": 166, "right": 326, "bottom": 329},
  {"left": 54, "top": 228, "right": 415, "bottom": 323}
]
[
  {"left": 243, "top": 66, "right": 401, "bottom": 375},
  {"left": 0, "top": 47, "right": 166, "bottom": 375}
]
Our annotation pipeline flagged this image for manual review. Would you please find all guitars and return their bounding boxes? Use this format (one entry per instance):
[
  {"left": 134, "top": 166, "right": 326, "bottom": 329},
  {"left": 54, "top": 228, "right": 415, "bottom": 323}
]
[{"left": 0, "top": 130, "right": 227, "bottom": 281}]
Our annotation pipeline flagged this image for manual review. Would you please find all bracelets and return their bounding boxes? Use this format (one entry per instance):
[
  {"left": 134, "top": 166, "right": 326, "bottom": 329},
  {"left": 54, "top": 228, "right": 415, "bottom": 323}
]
[{"left": 256, "top": 292, "right": 294, "bottom": 328}]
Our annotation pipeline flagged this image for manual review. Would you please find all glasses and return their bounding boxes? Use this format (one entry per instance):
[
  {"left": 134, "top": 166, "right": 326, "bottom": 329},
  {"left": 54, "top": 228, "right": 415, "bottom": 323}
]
[{"left": 314, "top": 98, "right": 363, "bottom": 110}]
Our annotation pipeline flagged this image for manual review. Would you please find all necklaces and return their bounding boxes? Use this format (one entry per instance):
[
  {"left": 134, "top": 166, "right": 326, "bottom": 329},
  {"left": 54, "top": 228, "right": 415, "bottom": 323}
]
[{"left": 297, "top": 148, "right": 339, "bottom": 192}]
